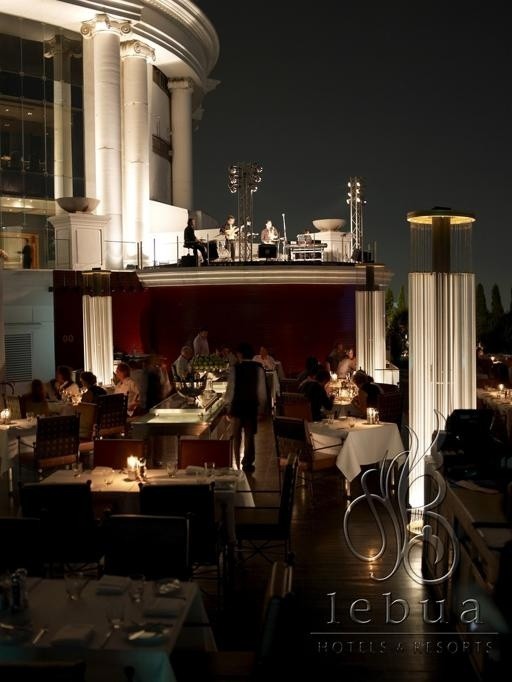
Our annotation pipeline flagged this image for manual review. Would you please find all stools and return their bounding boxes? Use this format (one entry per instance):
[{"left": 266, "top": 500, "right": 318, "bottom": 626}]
[{"left": 184, "top": 244, "right": 198, "bottom": 267}]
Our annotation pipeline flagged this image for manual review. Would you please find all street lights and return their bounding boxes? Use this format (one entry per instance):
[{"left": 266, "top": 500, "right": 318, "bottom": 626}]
[
  {"left": 227, "top": 160, "right": 265, "bottom": 263},
  {"left": 346, "top": 176, "right": 369, "bottom": 264},
  {"left": 406, "top": 206, "right": 479, "bottom": 539}
]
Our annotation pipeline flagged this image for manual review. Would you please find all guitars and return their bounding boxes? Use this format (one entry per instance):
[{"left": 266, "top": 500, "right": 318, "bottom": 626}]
[{"left": 225, "top": 217, "right": 249, "bottom": 239}]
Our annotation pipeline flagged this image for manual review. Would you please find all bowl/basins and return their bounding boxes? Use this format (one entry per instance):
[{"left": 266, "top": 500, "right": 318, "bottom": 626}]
[{"left": 172, "top": 380, "right": 205, "bottom": 398}]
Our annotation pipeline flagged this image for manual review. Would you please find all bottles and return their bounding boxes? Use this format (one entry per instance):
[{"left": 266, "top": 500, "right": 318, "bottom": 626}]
[{"left": 191, "top": 353, "right": 230, "bottom": 370}]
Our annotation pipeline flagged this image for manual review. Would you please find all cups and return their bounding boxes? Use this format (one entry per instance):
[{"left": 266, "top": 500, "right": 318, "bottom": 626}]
[
  {"left": 482, "top": 382, "right": 511, "bottom": 405},
  {"left": 316, "top": 406, "right": 382, "bottom": 430},
  {"left": 0, "top": 567, "right": 190, "bottom": 651},
  {"left": 70, "top": 460, "right": 242, "bottom": 481},
  {"left": 1, "top": 386, "right": 87, "bottom": 424}
]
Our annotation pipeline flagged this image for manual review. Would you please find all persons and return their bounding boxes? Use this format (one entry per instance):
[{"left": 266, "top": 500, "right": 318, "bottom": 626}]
[
  {"left": 46, "top": 362, "right": 141, "bottom": 419},
  {"left": 260, "top": 220, "right": 278, "bottom": 245},
  {"left": 169, "top": 328, "right": 384, "bottom": 423},
  {"left": 223, "top": 341, "right": 267, "bottom": 478},
  {"left": 220, "top": 215, "right": 239, "bottom": 262},
  {"left": 184, "top": 218, "right": 208, "bottom": 264},
  {"left": 21, "top": 238, "right": 32, "bottom": 270}
]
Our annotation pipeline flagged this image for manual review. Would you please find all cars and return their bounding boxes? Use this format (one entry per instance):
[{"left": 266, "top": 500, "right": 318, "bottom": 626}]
[{"left": 477, "top": 346, "right": 510, "bottom": 363}]
